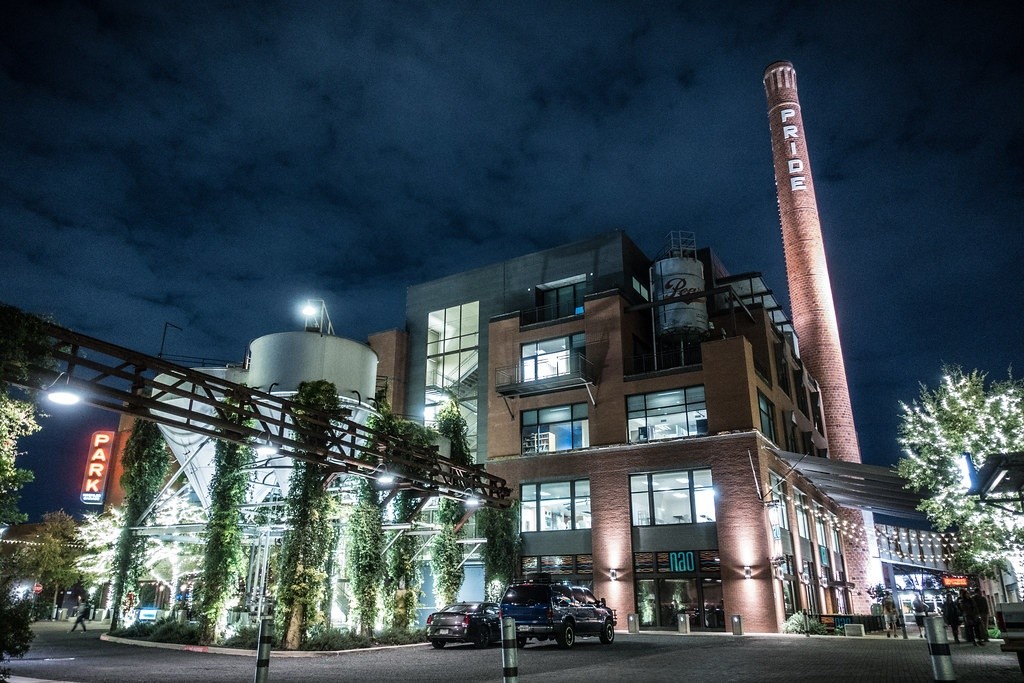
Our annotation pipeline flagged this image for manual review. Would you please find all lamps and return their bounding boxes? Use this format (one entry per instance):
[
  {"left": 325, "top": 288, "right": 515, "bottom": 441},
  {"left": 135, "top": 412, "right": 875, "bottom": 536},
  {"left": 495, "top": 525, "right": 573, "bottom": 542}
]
[{"left": 744, "top": 566, "right": 752, "bottom": 580}]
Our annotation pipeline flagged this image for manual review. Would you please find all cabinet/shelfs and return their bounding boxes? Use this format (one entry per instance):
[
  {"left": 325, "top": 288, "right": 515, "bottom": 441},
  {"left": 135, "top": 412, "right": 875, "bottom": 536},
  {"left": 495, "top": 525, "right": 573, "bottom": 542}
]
[{"left": 524, "top": 432, "right": 555, "bottom": 453}]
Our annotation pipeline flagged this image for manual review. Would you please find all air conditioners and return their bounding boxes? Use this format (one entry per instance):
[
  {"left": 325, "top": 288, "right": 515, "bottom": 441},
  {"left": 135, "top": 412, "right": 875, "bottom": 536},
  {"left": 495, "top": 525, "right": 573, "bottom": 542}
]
[
  {"left": 775, "top": 566, "right": 784, "bottom": 581},
  {"left": 821, "top": 578, "right": 828, "bottom": 589},
  {"left": 799, "top": 572, "right": 810, "bottom": 584}
]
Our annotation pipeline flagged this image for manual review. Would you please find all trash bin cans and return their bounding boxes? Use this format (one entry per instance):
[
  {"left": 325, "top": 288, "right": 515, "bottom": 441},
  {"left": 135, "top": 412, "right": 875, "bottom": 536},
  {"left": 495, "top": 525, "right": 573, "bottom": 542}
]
[
  {"left": 677, "top": 613, "right": 690, "bottom": 633},
  {"left": 627, "top": 613, "right": 639, "bottom": 633},
  {"left": 57, "top": 608, "right": 68, "bottom": 621},
  {"left": 732, "top": 614, "right": 743, "bottom": 635}
]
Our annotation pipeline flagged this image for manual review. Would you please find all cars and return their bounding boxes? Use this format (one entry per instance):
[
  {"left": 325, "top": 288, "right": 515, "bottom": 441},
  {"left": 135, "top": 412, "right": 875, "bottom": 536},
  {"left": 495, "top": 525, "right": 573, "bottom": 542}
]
[{"left": 425, "top": 601, "right": 501, "bottom": 649}]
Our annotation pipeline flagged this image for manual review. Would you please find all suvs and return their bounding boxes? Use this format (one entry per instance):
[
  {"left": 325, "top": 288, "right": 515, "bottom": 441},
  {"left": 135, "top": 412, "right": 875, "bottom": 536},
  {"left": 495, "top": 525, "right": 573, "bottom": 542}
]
[{"left": 499, "top": 579, "right": 617, "bottom": 649}]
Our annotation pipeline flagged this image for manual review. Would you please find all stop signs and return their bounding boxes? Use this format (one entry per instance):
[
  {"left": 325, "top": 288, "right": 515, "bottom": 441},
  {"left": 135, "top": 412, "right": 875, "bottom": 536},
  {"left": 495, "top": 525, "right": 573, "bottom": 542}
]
[{"left": 34, "top": 584, "right": 43, "bottom": 593}]
